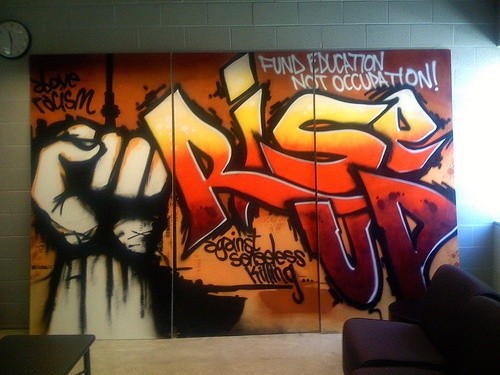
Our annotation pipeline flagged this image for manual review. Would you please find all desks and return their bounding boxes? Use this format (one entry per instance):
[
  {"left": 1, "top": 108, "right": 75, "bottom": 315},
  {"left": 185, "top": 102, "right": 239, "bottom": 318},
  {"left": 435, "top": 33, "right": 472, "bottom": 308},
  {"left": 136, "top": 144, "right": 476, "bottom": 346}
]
[{"left": 1, "top": 334, "right": 96, "bottom": 374}]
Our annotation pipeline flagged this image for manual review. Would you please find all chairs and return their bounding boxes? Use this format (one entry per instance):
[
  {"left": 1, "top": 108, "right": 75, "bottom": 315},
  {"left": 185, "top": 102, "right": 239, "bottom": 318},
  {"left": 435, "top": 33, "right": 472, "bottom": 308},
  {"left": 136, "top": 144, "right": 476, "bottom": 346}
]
[
  {"left": 342, "top": 263, "right": 499, "bottom": 375},
  {"left": 345, "top": 295, "right": 499, "bottom": 374}
]
